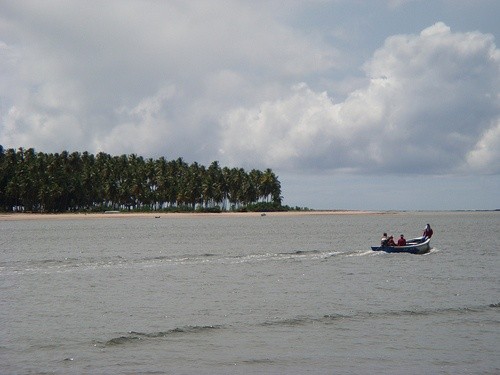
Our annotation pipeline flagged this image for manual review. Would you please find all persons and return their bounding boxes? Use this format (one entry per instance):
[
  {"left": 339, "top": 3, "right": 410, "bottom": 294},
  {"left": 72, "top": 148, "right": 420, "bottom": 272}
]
[
  {"left": 380, "top": 232, "right": 388, "bottom": 247},
  {"left": 420, "top": 223, "right": 433, "bottom": 242},
  {"left": 397, "top": 234, "right": 407, "bottom": 246},
  {"left": 387, "top": 235, "right": 397, "bottom": 246}
]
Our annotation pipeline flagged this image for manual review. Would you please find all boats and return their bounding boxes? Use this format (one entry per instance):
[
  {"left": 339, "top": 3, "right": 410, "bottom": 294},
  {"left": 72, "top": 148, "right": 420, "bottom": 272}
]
[{"left": 370, "top": 236, "right": 430, "bottom": 253}]
